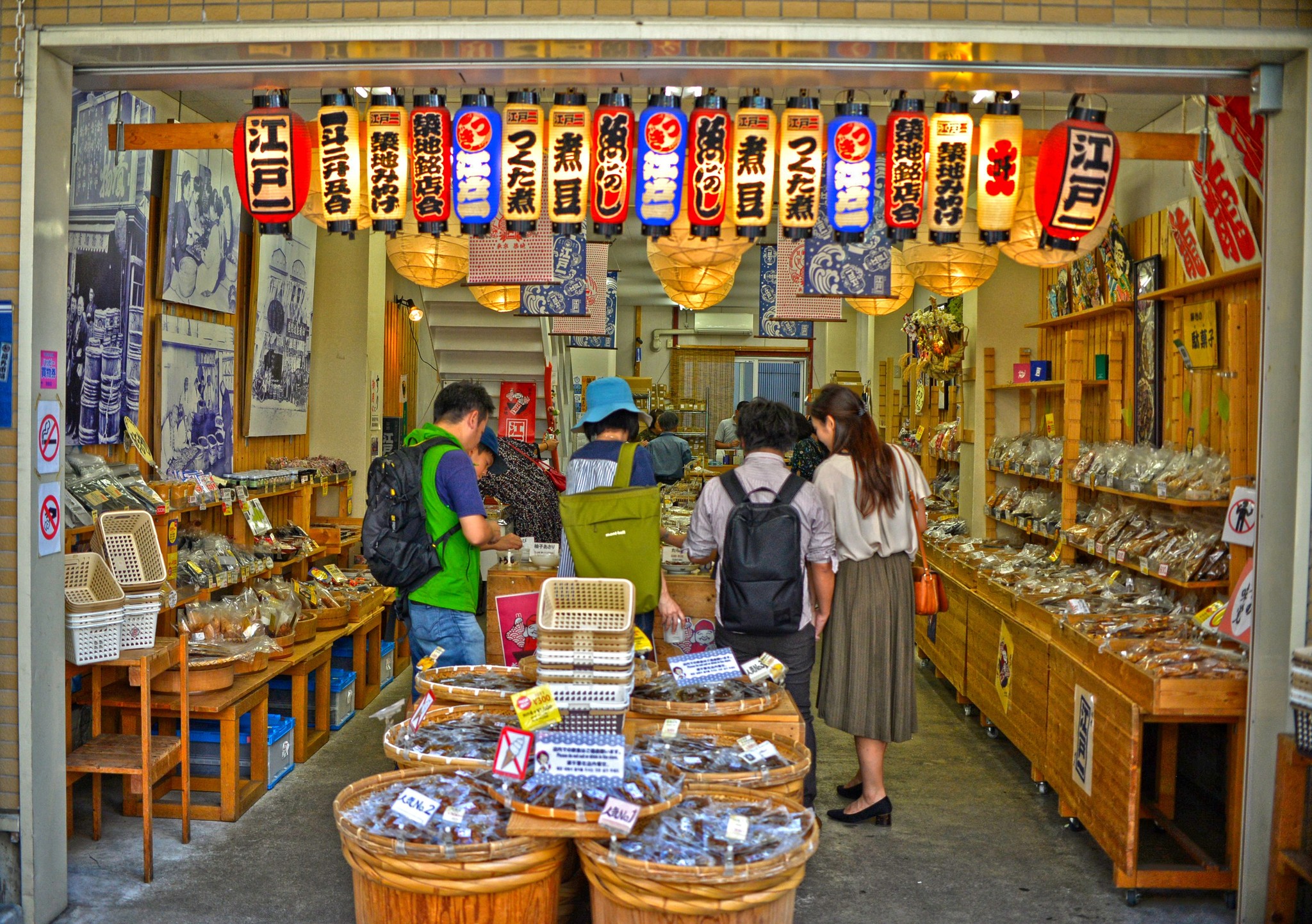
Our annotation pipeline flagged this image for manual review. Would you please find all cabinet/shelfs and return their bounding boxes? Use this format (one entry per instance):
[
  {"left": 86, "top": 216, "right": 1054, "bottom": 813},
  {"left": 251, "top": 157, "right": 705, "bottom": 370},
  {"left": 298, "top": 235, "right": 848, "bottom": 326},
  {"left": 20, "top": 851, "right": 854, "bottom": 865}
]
[
  {"left": 886, "top": 254, "right": 1262, "bottom": 905},
  {"left": 66, "top": 465, "right": 411, "bottom": 886},
  {"left": 630, "top": 387, "right": 710, "bottom": 461}
]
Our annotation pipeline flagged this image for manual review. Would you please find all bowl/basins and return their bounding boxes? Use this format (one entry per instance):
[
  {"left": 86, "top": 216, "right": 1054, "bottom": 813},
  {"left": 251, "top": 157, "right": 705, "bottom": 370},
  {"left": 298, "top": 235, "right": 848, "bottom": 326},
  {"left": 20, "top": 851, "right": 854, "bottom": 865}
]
[
  {"left": 530, "top": 555, "right": 560, "bottom": 569},
  {"left": 496, "top": 550, "right": 523, "bottom": 570}
]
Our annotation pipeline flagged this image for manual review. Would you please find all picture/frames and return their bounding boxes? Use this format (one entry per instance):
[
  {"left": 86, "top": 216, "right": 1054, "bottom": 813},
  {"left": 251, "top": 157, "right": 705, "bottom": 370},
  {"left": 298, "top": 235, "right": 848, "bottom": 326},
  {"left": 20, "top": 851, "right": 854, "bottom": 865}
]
[{"left": 905, "top": 303, "right": 949, "bottom": 411}]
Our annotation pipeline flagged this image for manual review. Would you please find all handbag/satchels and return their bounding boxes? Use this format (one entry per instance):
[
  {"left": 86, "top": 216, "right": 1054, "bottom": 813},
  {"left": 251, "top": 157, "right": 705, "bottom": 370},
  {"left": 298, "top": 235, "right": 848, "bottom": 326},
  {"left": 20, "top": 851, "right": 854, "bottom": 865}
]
[{"left": 913, "top": 560, "right": 950, "bottom": 618}]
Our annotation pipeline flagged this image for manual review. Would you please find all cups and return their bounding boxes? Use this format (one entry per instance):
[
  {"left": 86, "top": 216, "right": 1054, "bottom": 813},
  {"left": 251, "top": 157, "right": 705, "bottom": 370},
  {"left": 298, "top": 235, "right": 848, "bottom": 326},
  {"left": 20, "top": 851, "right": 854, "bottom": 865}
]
[{"left": 723, "top": 455, "right": 729, "bottom": 465}]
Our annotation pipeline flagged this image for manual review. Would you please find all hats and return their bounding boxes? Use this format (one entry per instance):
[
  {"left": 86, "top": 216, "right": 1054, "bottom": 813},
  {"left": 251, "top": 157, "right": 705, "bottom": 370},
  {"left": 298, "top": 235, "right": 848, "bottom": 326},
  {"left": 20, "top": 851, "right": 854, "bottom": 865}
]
[
  {"left": 568, "top": 376, "right": 653, "bottom": 435},
  {"left": 480, "top": 427, "right": 509, "bottom": 477}
]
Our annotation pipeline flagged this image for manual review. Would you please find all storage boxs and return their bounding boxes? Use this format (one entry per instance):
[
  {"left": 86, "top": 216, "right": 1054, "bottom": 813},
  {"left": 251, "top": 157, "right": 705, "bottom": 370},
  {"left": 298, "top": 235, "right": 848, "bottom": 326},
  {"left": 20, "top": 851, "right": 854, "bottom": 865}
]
[
  {"left": 809, "top": 369, "right": 864, "bottom": 403},
  {"left": 616, "top": 374, "right": 706, "bottom": 457}
]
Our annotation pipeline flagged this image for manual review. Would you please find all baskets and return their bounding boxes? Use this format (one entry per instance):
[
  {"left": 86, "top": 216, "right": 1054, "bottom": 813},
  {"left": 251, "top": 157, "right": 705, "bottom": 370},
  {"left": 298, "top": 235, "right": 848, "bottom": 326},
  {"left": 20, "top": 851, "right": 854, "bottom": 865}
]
[
  {"left": 533, "top": 576, "right": 636, "bottom": 735},
  {"left": 57, "top": 508, "right": 167, "bottom": 668}
]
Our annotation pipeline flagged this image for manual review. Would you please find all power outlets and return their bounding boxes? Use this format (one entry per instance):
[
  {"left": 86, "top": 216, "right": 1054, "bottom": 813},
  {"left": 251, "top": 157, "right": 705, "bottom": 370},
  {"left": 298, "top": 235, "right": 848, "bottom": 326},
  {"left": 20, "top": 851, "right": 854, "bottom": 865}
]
[
  {"left": 894, "top": 365, "right": 901, "bottom": 379},
  {"left": 667, "top": 339, "right": 673, "bottom": 348}
]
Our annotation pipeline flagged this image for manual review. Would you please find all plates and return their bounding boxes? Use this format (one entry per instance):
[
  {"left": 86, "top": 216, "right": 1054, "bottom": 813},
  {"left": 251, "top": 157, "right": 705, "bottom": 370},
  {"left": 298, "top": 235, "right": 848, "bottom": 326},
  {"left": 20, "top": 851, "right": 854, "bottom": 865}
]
[{"left": 660, "top": 561, "right": 700, "bottom": 575}]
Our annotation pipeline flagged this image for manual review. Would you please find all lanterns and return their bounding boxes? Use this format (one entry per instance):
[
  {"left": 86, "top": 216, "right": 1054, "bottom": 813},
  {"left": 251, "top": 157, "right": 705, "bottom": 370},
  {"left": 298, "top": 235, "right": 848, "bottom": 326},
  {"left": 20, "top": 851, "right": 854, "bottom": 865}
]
[
  {"left": 652, "top": 145, "right": 759, "bottom": 267},
  {"left": 644, "top": 234, "right": 746, "bottom": 292},
  {"left": 902, "top": 204, "right": 1000, "bottom": 298},
  {"left": 295, "top": 109, "right": 410, "bottom": 235},
  {"left": 843, "top": 245, "right": 916, "bottom": 317},
  {"left": 1034, "top": 94, "right": 1120, "bottom": 253},
  {"left": 310, "top": 89, "right": 1027, "bottom": 250},
  {"left": 237, "top": 37, "right": 1115, "bottom": 95},
  {"left": 468, "top": 284, "right": 524, "bottom": 315},
  {"left": 658, "top": 280, "right": 737, "bottom": 312},
  {"left": 232, "top": 93, "right": 312, "bottom": 234},
  {"left": 992, "top": 157, "right": 1118, "bottom": 270},
  {"left": 383, "top": 196, "right": 476, "bottom": 287}
]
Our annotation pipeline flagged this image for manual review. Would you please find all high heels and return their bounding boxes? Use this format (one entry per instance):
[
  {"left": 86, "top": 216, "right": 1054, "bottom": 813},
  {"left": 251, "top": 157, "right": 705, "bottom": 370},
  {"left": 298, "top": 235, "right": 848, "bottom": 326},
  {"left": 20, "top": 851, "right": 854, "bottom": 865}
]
[
  {"left": 827, "top": 797, "right": 892, "bottom": 827},
  {"left": 836, "top": 783, "right": 864, "bottom": 798}
]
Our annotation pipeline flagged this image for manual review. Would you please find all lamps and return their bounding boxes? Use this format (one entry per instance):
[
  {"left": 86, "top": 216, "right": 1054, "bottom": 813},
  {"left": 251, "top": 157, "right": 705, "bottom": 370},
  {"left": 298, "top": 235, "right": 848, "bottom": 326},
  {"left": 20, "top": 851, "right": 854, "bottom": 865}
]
[{"left": 398, "top": 299, "right": 425, "bottom": 322}]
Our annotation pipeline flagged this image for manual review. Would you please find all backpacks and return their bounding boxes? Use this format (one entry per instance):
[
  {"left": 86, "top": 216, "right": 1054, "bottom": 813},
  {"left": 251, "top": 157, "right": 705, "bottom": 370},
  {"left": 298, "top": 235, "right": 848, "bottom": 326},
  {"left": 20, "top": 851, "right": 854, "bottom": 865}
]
[
  {"left": 359, "top": 437, "right": 466, "bottom": 588},
  {"left": 719, "top": 469, "right": 808, "bottom": 636},
  {"left": 554, "top": 481, "right": 667, "bottom": 618}
]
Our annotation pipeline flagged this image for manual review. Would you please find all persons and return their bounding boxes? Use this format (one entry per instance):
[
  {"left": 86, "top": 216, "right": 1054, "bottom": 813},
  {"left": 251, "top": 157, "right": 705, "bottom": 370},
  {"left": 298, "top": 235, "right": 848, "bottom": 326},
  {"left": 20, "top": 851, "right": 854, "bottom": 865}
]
[
  {"left": 64, "top": 169, "right": 314, "bottom": 479},
  {"left": 400, "top": 377, "right": 504, "bottom": 707},
  {"left": 714, "top": 400, "right": 750, "bottom": 450},
  {"left": 789, "top": 410, "right": 824, "bottom": 481},
  {"left": 555, "top": 375, "right": 687, "bottom": 662},
  {"left": 806, "top": 382, "right": 934, "bottom": 828},
  {"left": 645, "top": 410, "right": 693, "bottom": 506},
  {"left": 475, "top": 436, "right": 563, "bottom": 545},
  {"left": 468, "top": 425, "right": 525, "bottom": 552},
  {"left": 638, "top": 408, "right": 664, "bottom": 444},
  {"left": 681, "top": 396, "right": 836, "bottom": 827}
]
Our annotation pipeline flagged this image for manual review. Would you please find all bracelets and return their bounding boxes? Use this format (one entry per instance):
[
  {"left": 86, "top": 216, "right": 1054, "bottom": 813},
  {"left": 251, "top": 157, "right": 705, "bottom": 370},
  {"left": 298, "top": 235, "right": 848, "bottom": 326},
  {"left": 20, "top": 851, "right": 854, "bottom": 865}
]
[
  {"left": 661, "top": 530, "right": 670, "bottom": 541},
  {"left": 545, "top": 442, "right": 549, "bottom": 451}
]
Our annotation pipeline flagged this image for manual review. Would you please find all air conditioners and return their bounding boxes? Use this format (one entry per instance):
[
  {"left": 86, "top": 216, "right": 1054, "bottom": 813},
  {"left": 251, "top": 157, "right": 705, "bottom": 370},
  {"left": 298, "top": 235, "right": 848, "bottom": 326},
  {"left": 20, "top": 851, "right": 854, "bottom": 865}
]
[{"left": 694, "top": 313, "right": 754, "bottom": 337}]
